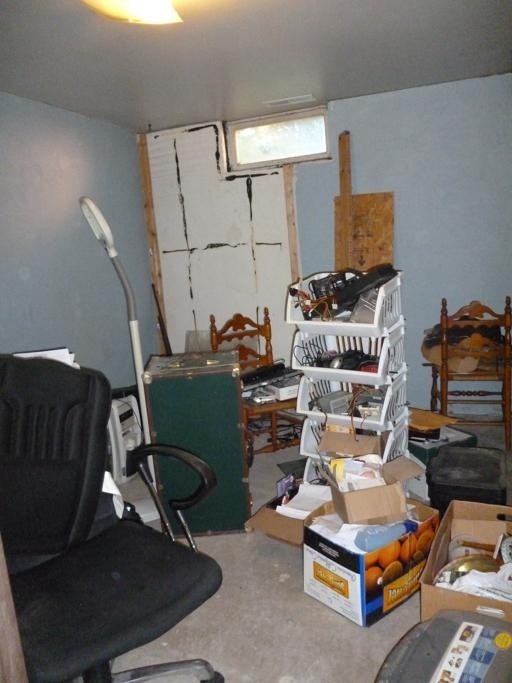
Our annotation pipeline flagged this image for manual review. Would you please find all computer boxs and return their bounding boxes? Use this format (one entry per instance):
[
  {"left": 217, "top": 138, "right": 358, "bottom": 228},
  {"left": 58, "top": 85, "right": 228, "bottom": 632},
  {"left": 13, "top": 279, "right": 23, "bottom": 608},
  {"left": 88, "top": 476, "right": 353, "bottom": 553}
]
[{"left": 106, "top": 395, "right": 142, "bottom": 486}]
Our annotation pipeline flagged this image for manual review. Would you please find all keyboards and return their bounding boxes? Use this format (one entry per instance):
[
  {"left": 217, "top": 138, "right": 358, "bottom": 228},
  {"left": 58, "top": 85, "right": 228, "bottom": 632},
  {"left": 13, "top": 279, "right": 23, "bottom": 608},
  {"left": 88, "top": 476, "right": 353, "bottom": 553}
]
[{"left": 239, "top": 366, "right": 303, "bottom": 392}]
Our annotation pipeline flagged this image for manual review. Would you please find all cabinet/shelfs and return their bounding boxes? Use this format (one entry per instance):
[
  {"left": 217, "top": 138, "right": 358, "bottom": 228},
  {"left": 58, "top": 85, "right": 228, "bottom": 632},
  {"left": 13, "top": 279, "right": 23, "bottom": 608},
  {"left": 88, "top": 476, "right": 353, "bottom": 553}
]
[{"left": 286, "top": 271, "right": 412, "bottom": 486}]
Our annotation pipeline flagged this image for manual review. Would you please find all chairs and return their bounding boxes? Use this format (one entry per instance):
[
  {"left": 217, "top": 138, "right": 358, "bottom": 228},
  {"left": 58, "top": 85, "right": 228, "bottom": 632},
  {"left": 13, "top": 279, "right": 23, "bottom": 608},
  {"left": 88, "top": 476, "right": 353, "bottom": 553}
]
[
  {"left": 431, "top": 296, "right": 512, "bottom": 450},
  {"left": 1, "top": 355, "right": 224, "bottom": 683},
  {"left": 209, "top": 306, "right": 305, "bottom": 452}
]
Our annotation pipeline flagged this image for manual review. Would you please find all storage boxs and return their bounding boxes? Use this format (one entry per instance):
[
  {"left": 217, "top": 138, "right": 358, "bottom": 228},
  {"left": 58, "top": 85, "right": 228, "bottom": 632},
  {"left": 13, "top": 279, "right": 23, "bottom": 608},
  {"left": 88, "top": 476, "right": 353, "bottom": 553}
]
[
  {"left": 374, "top": 609, "right": 512, "bottom": 683},
  {"left": 304, "top": 498, "right": 440, "bottom": 627},
  {"left": 245, "top": 495, "right": 304, "bottom": 547},
  {"left": 417, "top": 500, "right": 512, "bottom": 624},
  {"left": 275, "top": 425, "right": 508, "bottom": 523}
]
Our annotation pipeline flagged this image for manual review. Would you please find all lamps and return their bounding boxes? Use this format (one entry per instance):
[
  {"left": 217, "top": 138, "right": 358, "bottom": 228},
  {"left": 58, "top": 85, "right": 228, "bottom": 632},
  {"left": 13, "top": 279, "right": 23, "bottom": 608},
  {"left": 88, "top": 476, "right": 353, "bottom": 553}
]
[{"left": 79, "top": 195, "right": 156, "bottom": 493}]
